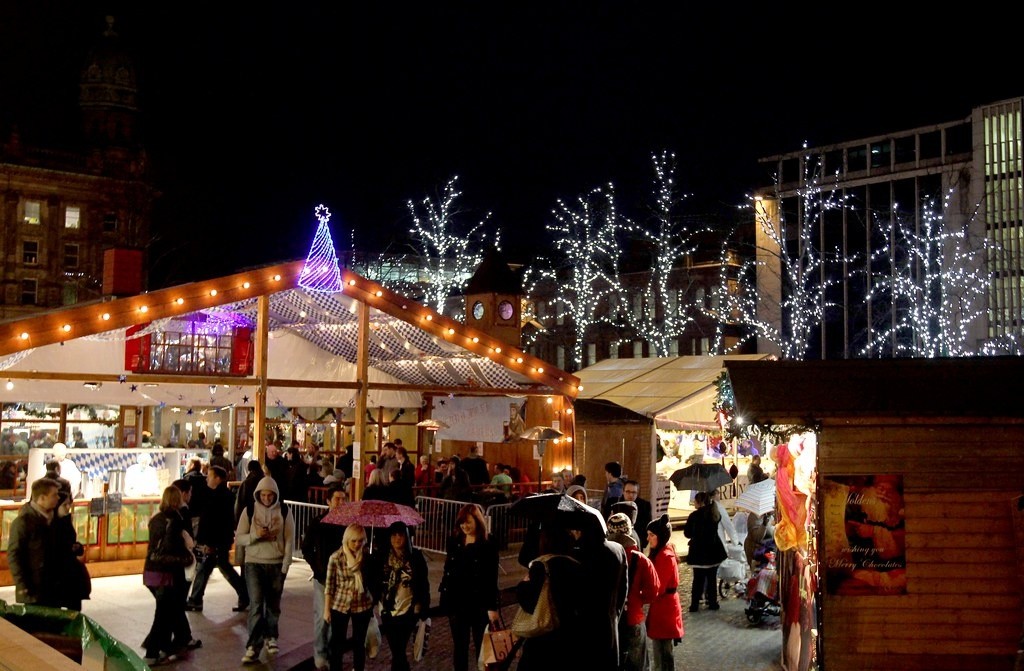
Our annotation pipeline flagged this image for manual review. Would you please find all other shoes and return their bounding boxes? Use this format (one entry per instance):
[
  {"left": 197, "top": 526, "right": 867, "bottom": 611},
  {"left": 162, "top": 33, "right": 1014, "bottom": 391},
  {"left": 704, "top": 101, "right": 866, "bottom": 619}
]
[
  {"left": 233, "top": 604, "right": 248, "bottom": 611},
  {"left": 186, "top": 604, "right": 203, "bottom": 612},
  {"left": 173, "top": 638, "right": 201, "bottom": 648},
  {"left": 689, "top": 605, "right": 698, "bottom": 612},
  {"left": 143, "top": 656, "right": 169, "bottom": 666},
  {"left": 314, "top": 651, "right": 330, "bottom": 671},
  {"left": 709, "top": 602, "right": 720, "bottom": 610}
]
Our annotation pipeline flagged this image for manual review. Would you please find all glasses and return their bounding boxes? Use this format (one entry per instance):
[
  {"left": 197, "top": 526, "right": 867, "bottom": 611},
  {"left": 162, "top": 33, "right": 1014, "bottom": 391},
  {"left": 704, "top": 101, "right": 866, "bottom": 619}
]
[{"left": 626, "top": 490, "right": 636, "bottom": 494}]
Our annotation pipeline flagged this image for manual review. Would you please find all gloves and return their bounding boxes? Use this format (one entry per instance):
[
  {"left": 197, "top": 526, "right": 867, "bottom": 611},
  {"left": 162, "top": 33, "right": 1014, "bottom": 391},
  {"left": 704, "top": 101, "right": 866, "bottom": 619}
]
[{"left": 763, "top": 515, "right": 771, "bottom": 526}]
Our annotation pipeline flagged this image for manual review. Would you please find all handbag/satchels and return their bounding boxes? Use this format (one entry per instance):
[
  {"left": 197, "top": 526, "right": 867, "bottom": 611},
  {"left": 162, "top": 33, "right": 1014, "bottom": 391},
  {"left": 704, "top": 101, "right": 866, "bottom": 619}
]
[
  {"left": 478, "top": 602, "right": 525, "bottom": 671},
  {"left": 365, "top": 614, "right": 382, "bottom": 660},
  {"left": 619, "top": 604, "right": 629, "bottom": 651},
  {"left": 413, "top": 617, "right": 432, "bottom": 662},
  {"left": 142, "top": 560, "right": 174, "bottom": 587},
  {"left": 512, "top": 561, "right": 559, "bottom": 638}
]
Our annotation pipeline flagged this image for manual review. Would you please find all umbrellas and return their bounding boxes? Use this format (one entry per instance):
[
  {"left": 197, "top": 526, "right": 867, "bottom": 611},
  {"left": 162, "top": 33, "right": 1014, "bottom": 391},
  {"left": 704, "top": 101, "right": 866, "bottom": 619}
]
[
  {"left": 320, "top": 500, "right": 426, "bottom": 528},
  {"left": 733, "top": 478, "right": 776, "bottom": 518},
  {"left": 669, "top": 463, "right": 733, "bottom": 492}
]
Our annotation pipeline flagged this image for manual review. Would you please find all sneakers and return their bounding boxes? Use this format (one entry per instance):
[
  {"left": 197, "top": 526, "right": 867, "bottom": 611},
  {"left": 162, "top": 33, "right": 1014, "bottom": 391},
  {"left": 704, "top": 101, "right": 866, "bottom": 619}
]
[
  {"left": 242, "top": 645, "right": 260, "bottom": 663},
  {"left": 266, "top": 637, "right": 279, "bottom": 654}
]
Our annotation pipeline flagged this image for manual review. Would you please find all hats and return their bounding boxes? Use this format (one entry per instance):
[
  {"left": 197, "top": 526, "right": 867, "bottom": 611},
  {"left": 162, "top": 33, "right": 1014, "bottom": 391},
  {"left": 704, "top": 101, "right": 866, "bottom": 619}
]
[
  {"left": 647, "top": 514, "right": 672, "bottom": 559},
  {"left": 607, "top": 512, "right": 632, "bottom": 535},
  {"left": 451, "top": 455, "right": 461, "bottom": 464}
]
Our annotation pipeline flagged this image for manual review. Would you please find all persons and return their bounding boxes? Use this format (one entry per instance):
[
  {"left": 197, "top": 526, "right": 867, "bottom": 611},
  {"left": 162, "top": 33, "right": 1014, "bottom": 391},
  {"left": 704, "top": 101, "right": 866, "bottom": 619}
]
[
  {"left": 0, "top": 458, "right": 91, "bottom": 611},
  {"left": 231, "top": 461, "right": 296, "bottom": 664},
  {"left": 265, "top": 438, "right": 532, "bottom": 555},
  {"left": 689, "top": 447, "right": 704, "bottom": 506},
  {"left": 142, "top": 481, "right": 203, "bottom": 657},
  {"left": 1, "top": 427, "right": 88, "bottom": 455},
  {"left": 183, "top": 432, "right": 252, "bottom": 612},
  {"left": 370, "top": 522, "right": 431, "bottom": 671},
  {"left": 729, "top": 461, "right": 739, "bottom": 496},
  {"left": 438, "top": 505, "right": 500, "bottom": 671},
  {"left": 325, "top": 526, "right": 374, "bottom": 671},
  {"left": 744, "top": 454, "right": 783, "bottom": 629},
  {"left": 510, "top": 461, "right": 686, "bottom": 671},
  {"left": 683, "top": 490, "right": 739, "bottom": 612},
  {"left": 303, "top": 488, "right": 348, "bottom": 671},
  {"left": 740, "top": 439, "right": 758, "bottom": 456},
  {"left": 142, "top": 428, "right": 164, "bottom": 449}
]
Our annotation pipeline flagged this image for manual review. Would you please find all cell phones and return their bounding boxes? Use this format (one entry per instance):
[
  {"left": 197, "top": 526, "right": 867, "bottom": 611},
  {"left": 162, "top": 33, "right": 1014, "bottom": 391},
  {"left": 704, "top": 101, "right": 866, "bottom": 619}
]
[{"left": 263, "top": 526, "right": 269, "bottom": 531}]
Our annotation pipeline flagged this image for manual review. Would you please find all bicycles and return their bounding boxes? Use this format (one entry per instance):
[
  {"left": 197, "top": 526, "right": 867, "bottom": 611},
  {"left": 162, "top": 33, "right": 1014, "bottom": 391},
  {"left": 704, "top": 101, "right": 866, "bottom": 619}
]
[{"left": 717, "top": 541, "right": 781, "bottom": 624}]
